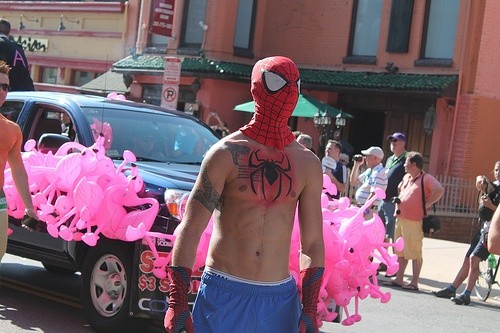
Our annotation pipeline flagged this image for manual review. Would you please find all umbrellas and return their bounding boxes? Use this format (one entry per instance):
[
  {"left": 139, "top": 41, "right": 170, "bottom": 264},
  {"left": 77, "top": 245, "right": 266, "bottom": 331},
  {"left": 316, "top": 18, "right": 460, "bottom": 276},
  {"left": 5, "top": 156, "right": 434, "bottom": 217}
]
[{"left": 233, "top": 94, "right": 354, "bottom": 119}]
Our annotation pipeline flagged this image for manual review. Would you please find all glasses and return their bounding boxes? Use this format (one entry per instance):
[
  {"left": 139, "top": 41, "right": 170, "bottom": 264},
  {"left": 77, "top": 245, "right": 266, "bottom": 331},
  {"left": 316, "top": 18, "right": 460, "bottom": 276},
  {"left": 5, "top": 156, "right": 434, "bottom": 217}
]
[
  {"left": 0, "top": 83, "right": 10, "bottom": 90},
  {"left": 391, "top": 139, "right": 398, "bottom": 142}
]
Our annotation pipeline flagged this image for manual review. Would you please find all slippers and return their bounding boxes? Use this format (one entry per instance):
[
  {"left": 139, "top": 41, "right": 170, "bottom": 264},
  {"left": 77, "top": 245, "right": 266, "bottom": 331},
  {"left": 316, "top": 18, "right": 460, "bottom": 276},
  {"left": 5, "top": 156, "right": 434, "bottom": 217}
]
[
  {"left": 382, "top": 281, "right": 402, "bottom": 287},
  {"left": 402, "top": 283, "right": 419, "bottom": 290}
]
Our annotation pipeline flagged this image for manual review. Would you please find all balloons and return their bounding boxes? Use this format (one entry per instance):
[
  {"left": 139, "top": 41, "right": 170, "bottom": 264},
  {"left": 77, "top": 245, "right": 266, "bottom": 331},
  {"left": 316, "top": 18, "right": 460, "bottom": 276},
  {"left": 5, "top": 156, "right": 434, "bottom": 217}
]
[{"left": 5, "top": 130, "right": 404, "bottom": 328}]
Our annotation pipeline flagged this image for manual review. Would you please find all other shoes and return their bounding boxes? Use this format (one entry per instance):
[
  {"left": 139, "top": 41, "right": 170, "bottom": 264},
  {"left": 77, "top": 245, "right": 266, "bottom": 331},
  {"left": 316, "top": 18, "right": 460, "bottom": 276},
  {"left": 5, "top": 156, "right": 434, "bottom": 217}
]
[
  {"left": 432, "top": 288, "right": 456, "bottom": 299},
  {"left": 451, "top": 294, "right": 470, "bottom": 306}
]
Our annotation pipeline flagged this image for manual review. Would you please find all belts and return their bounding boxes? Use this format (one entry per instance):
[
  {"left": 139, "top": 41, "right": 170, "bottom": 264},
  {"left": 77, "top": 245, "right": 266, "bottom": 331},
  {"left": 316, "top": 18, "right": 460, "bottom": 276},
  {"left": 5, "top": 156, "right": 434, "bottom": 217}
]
[{"left": 357, "top": 204, "right": 378, "bottom": 209}]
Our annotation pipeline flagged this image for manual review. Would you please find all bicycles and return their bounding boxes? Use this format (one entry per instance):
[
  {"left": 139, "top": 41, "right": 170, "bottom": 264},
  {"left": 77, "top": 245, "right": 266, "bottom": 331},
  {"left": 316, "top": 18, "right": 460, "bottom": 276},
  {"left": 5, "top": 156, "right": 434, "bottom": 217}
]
[{"left": 474, "top": 254, "right": 500, "bottom": 303}]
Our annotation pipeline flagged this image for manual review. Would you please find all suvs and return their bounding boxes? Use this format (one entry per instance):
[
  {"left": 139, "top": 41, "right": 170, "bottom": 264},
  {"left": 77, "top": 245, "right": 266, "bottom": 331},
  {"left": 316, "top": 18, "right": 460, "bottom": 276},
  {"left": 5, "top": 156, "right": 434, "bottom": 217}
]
[{"left": 0, "top": 91, "right": 221, "bottom": 333}]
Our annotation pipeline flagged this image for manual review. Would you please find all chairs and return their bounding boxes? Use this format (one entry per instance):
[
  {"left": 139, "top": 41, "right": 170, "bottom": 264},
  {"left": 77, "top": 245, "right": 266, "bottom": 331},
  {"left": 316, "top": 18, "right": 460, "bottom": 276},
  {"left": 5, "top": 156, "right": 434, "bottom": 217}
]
[
  {"left": 37, "top": 132, "right": 71, "bottom": 154},
  {"left": 472, "top": 254, "right": 500, "bottom": 302}
]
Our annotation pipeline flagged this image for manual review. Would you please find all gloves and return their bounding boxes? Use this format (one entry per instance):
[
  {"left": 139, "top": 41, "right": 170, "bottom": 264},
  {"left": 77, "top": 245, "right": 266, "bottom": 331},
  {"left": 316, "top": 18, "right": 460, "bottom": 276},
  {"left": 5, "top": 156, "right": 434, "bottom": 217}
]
[
  {"left": 298, "top": 266, "right": 324, "bottom": 333},
  {"left": 164, "top": 266, "right": 194, "bottom": 333}
]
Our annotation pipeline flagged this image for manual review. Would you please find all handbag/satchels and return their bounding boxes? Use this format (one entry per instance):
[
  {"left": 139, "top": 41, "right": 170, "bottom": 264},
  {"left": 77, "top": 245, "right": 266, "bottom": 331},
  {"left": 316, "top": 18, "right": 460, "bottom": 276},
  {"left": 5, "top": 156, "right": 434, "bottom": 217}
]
[{"left": 422, "top": 215, "right": 440, "bottom": 233}]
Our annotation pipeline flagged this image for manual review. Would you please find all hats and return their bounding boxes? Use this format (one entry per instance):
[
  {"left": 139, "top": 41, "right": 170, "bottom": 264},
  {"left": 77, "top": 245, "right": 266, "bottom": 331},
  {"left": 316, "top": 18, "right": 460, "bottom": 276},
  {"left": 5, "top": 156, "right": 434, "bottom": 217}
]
[
  {"left": 361, "top": 147, "right": 384, "bottom": 159},
  {"left": 388, "top": 133, "right": 405, "bottom": 141}
]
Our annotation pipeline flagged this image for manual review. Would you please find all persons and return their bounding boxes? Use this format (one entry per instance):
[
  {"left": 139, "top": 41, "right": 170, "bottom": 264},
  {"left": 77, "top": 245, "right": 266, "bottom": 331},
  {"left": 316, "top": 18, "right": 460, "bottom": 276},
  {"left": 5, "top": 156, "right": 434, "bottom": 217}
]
[
  {"left": 161, "top": 57, "right": 325, "bottom": 333},
  {"left": 292, "top": 131, "right": 500, "bottom": 307},
  {"left": 0, "top": 21, "right": 37, "bottom": 260}
]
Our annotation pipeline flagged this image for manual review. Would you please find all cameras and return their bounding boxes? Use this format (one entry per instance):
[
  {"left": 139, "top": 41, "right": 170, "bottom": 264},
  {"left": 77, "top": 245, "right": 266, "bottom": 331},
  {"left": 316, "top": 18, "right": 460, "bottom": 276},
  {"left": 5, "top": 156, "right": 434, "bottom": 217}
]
[
  {"left": 352, "top": 155, "right": 363, "bottom": 162},
  {"left": 391, "top": 197, "right": 400, "bottom": 204}
]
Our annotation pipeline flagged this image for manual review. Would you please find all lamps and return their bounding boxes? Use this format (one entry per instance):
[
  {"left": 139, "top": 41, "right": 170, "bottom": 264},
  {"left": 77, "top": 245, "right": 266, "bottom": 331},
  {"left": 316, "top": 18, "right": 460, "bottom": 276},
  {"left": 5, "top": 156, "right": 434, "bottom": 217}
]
[{"left": 15, "top": 14, "right": 81, "bottom": 34}]
